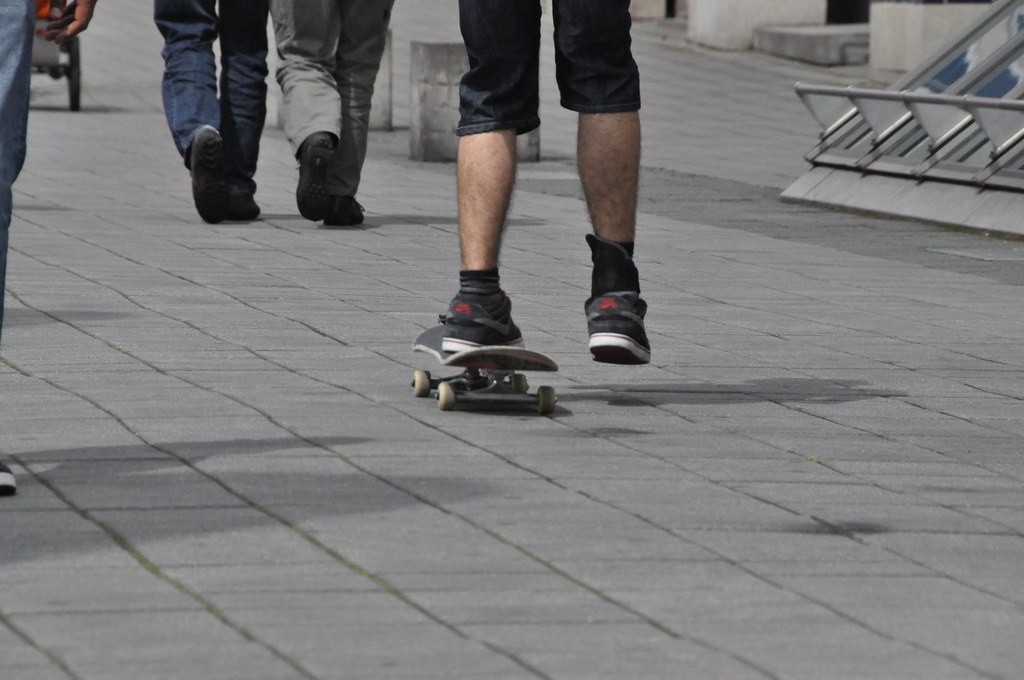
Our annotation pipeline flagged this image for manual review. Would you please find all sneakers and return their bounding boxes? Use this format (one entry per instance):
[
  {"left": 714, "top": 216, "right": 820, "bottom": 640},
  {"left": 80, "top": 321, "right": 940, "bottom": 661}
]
[
  {"left": 439, "top": 290, "right": 525, "bottom": 351},
  {"left": 585, "top": 291, "right": 651, "bottom": 364}
]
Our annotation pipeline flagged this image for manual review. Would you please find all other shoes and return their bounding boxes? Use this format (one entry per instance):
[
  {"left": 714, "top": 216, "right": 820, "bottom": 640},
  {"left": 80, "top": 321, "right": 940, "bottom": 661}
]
[
  {"left": 296, "top": 131, "right": 340, "bottom": 221},
  {"left": 190, "top": 124, "right": 228, "bottom": 225},
  {"left": 324, "top": 200, "right": 364, "bottom": 227},
  {"left": 222, "top": 188, "right": 260, "bottom": 221}
]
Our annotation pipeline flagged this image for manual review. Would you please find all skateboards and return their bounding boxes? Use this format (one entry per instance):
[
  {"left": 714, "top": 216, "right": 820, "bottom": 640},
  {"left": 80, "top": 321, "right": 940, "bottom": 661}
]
[{"left": 409, "top": 324, "right": 560, "bottom": 415}]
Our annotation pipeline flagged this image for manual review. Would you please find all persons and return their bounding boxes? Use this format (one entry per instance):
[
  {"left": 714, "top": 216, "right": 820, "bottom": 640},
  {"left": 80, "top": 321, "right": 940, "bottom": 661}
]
[
  {"left": 443, "top": 0, "right": 648, "bottom": 363},
  {"left": 152, "top": 0, "right": 270, "bottom": 221},
  {"left": 269, "top": 0, "right": 394, "bottom": 224},
  {"left": 0, "top": 0, "right": 96, "bottom": 496}
]
